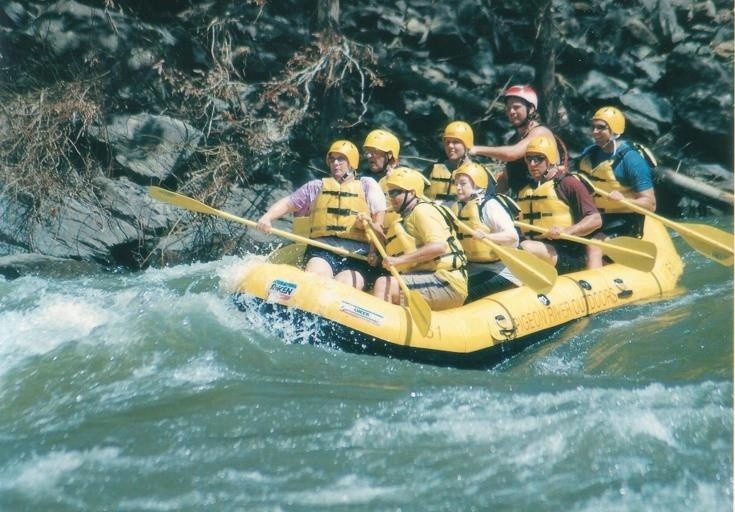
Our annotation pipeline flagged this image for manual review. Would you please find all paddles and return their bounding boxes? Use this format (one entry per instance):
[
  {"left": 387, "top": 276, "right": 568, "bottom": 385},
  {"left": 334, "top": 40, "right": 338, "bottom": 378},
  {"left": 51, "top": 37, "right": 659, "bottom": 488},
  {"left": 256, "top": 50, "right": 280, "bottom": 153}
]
[
  {"left": 515, "top": 222, "right": 657, "bottom": 272},
  {"left": 362, "top": 222, "right": 432, "bottom": 338},
  {"left": 421, "top": 194, "right": 558, "bottom": 294},
  {"left": 149, "top": 186, "right": 374, "bottom": 261},
  {"left": 595, "top": 188, "right": 734, "bottom": 267}
]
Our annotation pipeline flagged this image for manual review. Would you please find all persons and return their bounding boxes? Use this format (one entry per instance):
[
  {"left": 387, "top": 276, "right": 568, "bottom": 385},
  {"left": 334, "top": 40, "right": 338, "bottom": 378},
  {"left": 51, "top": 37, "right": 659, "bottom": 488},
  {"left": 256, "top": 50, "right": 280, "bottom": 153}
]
[
  {"left": 447, "top": 163, "right": 523, "bottom": 301},
  {"left": 255, "top": 139, "right": 386, "bottom": 293},
  {"left": 356, "top": 168, "right": 467, "bottom": 311},
  {"left": 467, "top": 85, "right": 568, "bottom": 180},
  {"left": 419, "top": 121, "right": 499, "bottom": 207},
  {"left": 564, "top": 106, "right": 658, "bottom": 269},
  {"left": 358, "top": 129, "right": 403, "bottom": 182},
  {"left": 509, "top": 137, "right": 601, "bottom": 273}
]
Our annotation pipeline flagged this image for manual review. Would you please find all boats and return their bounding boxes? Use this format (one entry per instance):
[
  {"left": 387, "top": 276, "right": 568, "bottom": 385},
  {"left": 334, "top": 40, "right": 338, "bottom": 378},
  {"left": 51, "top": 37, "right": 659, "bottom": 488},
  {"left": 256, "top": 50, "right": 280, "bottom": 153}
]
[{"left": 232, "top": 215, "right": 685, "bottom": 369}]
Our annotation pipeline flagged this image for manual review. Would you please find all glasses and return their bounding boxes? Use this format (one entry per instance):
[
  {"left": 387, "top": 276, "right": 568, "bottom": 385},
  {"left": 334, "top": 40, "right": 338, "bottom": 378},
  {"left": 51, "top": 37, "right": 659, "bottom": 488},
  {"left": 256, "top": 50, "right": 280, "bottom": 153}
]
[
  {"left": 526, "top": 156, "right": 544, "bottom": 163},
  {"left": 388, "top": 189, "right": 403, "bottom": 196},
  {"left": 592, "top": 124, "right": 606, "bottom": 130},
  {"left": 328, "top": 156, "right": 346, "bottom": 162}
]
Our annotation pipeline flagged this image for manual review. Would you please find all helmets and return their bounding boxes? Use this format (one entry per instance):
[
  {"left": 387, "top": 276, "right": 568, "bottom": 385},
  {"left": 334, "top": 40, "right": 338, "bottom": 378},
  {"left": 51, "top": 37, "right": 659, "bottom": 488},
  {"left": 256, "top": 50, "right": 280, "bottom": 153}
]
[
  {"left": 443, "top": 121, "right": 473, "bottom": 149},
  {"left": 526, "top": 136, "right": 556, "bottom": 164},
  {"left": 326, "top": 140, "right": 360, "bottom": 170},
  {"left": 386, "top": 167, "right": 425, "bottom": 198},
  {"left": 593, "top": 106, "right": 625, "bottom": 134},
  {"left": 504, "top": 85, "right": 537, "bottom": 111},
  {"left": 364, "top": 130, "right": 399, "bottom": 161},
  {"left": 455, "top": 163, "right": 488, "bottom": 188}
]
